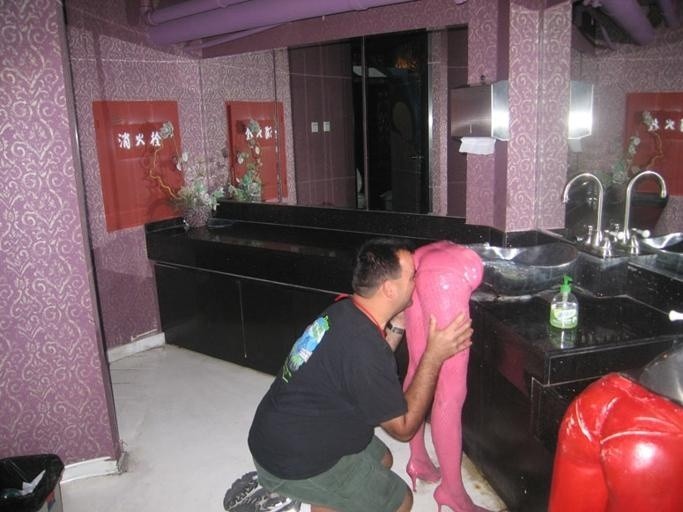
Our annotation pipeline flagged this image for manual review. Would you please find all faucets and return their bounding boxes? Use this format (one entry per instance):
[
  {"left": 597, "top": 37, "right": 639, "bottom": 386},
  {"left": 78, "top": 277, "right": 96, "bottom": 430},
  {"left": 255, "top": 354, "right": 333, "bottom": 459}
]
[
  {"left": 623, "top": 169, "right": 668, "bottom": 244},
  {"left": 561, "top": 173, "right": 603, "bottom": 232}
]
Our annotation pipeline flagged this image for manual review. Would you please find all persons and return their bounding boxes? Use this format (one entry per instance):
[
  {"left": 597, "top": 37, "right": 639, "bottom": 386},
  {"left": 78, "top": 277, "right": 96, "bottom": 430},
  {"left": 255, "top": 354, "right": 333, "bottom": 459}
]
[
  {"left": 223, "top": 240, "right": 475, "bottom": 512},
  {"left": 546, "top": 369, "right": 683, "bottom": 511},
  {"left": 402, "top": 241, "right": 484, "bottom": 512}
]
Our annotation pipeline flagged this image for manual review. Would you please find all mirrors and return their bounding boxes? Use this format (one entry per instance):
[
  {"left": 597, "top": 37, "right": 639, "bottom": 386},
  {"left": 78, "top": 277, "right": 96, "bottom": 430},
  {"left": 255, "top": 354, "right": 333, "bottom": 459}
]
[
  {"left": 534, "top": 0, "right": 683, "bottom": 284},
  {"left": 198, "top": 22, "right": 470, "bottom": 219}
]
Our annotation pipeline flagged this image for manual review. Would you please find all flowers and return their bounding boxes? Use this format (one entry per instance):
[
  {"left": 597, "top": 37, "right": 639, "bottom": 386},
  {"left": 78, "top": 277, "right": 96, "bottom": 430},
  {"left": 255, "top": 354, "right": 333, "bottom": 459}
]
[
  {"left": 599, "top": 111, "right": 664, "bottom": 189},
  {"left": 228, "top": 118, "right": 273, "bottom": 201},
  {"left": 149, "top": 121, "right": 225, "bottom": 211}
]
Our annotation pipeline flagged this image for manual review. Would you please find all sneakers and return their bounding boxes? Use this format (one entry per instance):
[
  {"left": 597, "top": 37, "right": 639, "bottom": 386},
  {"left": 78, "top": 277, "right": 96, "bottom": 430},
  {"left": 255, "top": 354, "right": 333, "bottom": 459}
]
[{"left": 224, "top": 471, "right": 301, "bottom": 512}]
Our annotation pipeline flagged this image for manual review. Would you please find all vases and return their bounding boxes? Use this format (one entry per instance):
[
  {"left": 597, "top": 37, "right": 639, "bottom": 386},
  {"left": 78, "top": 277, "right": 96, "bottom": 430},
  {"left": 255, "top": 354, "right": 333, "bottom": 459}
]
[
  {"left": 607, "top": 184, "right": 630, "bottom": 203},
  {"left": 182, "top": 207, "right": 210, "bottom": 228},
  {"left": 245, "top": 190, "right": 262, "bottom": 203}
]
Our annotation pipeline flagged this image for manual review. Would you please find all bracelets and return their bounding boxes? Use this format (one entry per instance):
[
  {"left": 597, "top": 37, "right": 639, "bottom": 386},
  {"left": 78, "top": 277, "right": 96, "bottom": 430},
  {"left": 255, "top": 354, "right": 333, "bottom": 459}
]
[{"left": 382, "top": 319, "right": 410, "bottom": 338}]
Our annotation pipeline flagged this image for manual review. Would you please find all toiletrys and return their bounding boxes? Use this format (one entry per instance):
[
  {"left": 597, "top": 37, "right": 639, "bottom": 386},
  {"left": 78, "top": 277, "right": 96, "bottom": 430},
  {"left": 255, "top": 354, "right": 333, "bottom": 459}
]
[{"left": 549, "top": 275, "right": 578, "bottom": 328}]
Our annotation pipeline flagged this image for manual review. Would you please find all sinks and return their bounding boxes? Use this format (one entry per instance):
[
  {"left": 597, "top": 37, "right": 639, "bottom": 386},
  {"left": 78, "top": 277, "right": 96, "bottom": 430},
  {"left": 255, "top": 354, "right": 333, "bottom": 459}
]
[
  {"left": 639, "top": 231, "right": 683, "bottom": 272},
  {"left": 462, "top": 242, "right": 579, "bottom": 298}
]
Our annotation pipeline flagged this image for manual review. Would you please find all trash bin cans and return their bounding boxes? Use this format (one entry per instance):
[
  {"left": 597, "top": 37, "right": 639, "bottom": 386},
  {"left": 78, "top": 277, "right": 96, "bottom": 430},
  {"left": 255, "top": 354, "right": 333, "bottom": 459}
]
[{"left": 0, "top": 454, "right": 65, "bottom": 512}]
